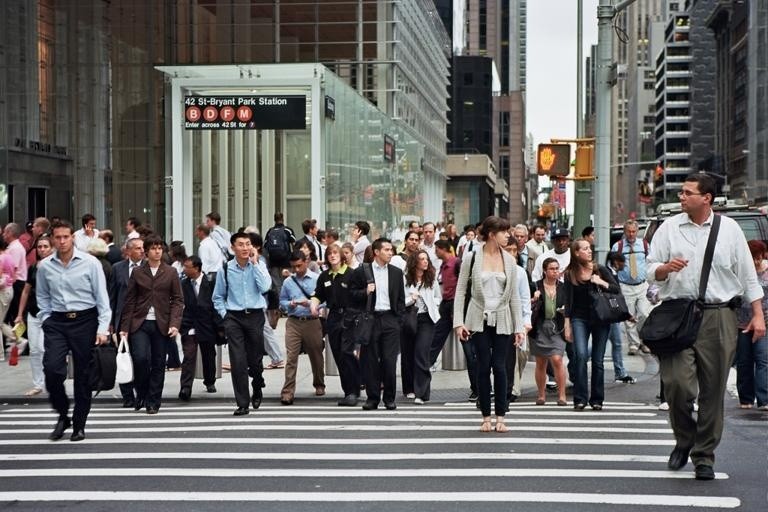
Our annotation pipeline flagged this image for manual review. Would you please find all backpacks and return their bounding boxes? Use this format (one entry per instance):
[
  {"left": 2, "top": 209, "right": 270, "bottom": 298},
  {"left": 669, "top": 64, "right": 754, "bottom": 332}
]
[{"left": 265, "top": 225, "right": 291, "bottom": 268}]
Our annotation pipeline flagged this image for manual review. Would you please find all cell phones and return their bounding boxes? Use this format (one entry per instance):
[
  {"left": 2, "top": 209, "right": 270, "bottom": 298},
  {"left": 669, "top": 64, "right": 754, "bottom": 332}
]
[
  {"left": 296, "top": 301, "right": 301, "bottom": 305},
  {"left": 249, "top": 244, "right": 253, "bottom": 256},
  {"left": 463, "top": 331, "right": 468, "bottom": 342}
]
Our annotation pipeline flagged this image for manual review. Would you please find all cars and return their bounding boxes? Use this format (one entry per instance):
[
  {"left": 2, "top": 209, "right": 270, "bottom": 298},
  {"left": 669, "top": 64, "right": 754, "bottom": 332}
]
[{"left": 610, "top": 196, "right": 767, "bottom": 258}]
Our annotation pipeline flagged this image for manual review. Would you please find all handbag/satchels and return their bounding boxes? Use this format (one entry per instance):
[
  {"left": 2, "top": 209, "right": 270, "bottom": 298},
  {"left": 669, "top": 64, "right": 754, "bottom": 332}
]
[
  {"left": 340, "top": 308, "right": 374, "bottom": 345},
  {"left": 593, "top": 291, "right": 631, "bottom": 324},
  {"left": 211, "top": 312, "right": 227, "bottom": 346},
  {"left": 401, "top": 305, "right": 417, "bottom": 336},
  {"left": 86, "top": 346, "right": 133, "bottom": 391},
  {"left": 638, "top": 298, "right": 703, "bottom": 354}
]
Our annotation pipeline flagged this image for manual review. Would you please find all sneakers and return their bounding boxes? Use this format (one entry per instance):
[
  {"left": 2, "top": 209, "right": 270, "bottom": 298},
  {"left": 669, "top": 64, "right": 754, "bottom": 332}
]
[
  {"left": 658, "top": 402, "right": 668, "bottom": 410},
  {"left": 616, "top": 375, "right": 636, "bottom": 384},
  {"left": 468, "top": 392, "right": 478, "bottom": 401},
  {"left": 695, "top": 465, "right": 714, "bottom": 479},
  {"left": 668, "top": 448, "right": 690, "bottom": 471}
]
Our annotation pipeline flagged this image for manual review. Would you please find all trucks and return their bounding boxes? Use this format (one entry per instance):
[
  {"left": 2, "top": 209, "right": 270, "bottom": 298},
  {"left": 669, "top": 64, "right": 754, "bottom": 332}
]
[{"left": 568, "top": 214, "right": 595, "bottom": 235}]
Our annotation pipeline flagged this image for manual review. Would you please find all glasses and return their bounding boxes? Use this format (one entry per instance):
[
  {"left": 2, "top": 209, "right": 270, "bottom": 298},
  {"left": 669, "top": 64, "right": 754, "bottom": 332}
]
[{"left": 677, "top": 192, "right": 700, "bottom": 196}]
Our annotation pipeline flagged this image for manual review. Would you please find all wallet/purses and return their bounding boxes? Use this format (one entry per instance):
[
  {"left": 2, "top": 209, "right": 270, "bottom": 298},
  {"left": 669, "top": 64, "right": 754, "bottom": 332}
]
[{"left": 11, "top": 321, "right": 25, "bottom": 340}]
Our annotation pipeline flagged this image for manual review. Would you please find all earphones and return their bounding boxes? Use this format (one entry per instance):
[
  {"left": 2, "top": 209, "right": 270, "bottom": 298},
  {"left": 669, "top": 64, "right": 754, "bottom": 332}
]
[
  {"left": 544, "top": 271, "right": 546, "bottom": 274},
  {"left": 492, "top": 235, "right": 495, "bottom": 239}
]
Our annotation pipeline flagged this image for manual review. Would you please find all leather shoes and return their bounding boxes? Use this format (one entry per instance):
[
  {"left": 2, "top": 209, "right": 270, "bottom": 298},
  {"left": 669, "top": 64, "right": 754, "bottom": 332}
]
[
  {"left": 338, "top": 393, "right": 395, "bottom": 410},
  {"left": 535, "top": 400, "right": 601, "bottom": 410},
  {"left": 123, "top": 400, "right": 158, "bottom": 413},
  {"left": 281, "top": 397, "right": 292, "bottom": 405},
  {"left": 407, "top": 393, "right": 423, "bottom": 405},
  {"left": 207, "top": 385, "right": 215, "bottom": 392},
  {"left": 315, "top": 388, "right": 324, "bottom": 395},
  {"left": 49, "top": 422, "right": 85, "bottom": 441},
  {"left": 233, "top": 388, "right": 262, "bottom": 415}
]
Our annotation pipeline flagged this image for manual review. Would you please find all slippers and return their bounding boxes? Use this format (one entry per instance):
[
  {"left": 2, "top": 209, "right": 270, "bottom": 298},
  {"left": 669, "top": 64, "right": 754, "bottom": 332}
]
[{"left": 479, "top": 421, "right": 505, "bottom": 433}]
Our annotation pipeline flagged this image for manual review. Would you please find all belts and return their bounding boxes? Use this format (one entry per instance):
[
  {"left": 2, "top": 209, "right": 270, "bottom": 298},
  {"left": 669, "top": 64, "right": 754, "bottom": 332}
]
[
  {"left": 52, "top": 306, "right": 96, "bottom": 319},
  {"left": 241, "top": 308, "right": 254, "bottom": 313},
  {"left": 289, "top": 315, "right": 317, "bottom": 322}
]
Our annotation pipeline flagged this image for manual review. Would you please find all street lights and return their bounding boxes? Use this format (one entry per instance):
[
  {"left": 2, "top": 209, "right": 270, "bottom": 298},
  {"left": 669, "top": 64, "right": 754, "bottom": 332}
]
[
  {"left": 698, "top": 170, "right": 727, "bottom": 198},
  {"left": 741, "top": 150, "right": 768, "bottom": 161}
]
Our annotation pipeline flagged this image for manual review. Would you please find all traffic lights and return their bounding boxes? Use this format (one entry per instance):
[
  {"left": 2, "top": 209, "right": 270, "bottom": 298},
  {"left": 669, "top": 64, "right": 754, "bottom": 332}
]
[
  {"left": 537, "top": 143, "right": 571, "bottom": 177},
  {"left": 537, "top": 210, "right": 544, "bottom": 219},
  {"left": 657, "top": 168, "right": 662, "bottom": 182}
]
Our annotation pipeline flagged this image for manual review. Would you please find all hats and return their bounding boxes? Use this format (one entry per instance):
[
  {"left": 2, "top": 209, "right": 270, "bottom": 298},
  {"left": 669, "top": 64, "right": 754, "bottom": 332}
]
[{"left": 551, "top": 228, "right": 567, "bottom": 238}]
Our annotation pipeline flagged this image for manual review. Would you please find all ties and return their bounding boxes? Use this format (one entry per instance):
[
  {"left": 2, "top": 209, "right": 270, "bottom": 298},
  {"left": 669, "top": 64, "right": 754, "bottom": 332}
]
[{"left": 629, "top": 243, "right": 636, "bottom": 280}]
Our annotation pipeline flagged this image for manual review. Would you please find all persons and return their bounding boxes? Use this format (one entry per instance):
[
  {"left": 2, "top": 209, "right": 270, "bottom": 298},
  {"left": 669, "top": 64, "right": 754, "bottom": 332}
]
[
  {"left": 1, "top": 210, "right": 654, "bottom": 441},
  {"left": 646, "top": 175, "right": 767, "bottom": 479}
]
